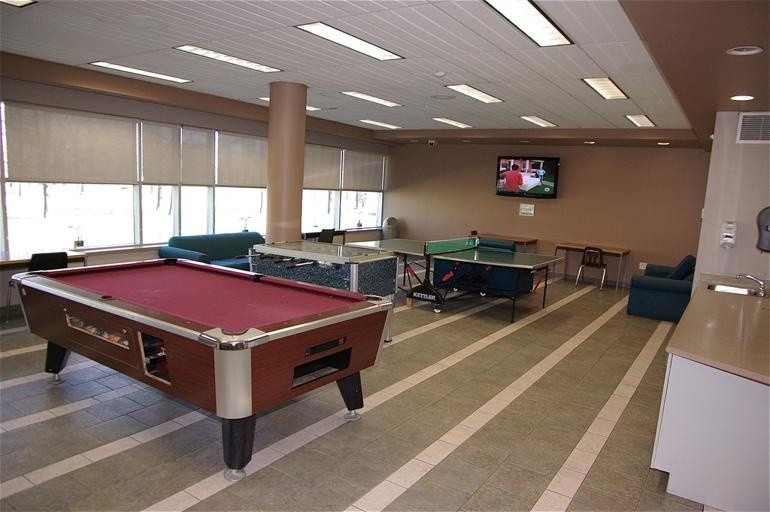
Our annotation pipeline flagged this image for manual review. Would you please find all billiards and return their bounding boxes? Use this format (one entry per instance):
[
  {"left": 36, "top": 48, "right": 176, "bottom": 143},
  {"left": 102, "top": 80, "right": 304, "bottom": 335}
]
[{"left": 69, "top": 316, "right": 128, "bottom": 346}]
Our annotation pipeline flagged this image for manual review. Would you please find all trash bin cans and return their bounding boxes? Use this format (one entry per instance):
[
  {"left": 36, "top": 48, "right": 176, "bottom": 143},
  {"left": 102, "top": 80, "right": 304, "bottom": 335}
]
[{"left": 382, "top": 217, "right": 399, "bottom": 239}]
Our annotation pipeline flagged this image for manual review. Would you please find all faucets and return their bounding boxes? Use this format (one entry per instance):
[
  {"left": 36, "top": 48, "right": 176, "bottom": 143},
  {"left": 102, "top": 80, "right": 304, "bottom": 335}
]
[{"left": 736, "top": 273, "right": 764, "bottom": 289}]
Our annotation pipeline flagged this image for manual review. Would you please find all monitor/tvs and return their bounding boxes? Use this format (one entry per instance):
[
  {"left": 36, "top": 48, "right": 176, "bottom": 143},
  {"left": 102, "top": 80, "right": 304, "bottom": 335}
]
[{"left": 495, "top": 155, "right": 560, "bottom": 199}]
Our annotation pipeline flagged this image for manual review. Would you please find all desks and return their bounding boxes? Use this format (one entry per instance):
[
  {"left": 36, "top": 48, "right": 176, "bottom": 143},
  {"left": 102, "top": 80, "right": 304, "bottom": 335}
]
[
  {"left": 1, "top": 251, "right": 87, "bottom": 266},
  {"left": 11, "top": 257, "right": 394, "bottom": 483},
  {"left": 344, "top": 233, "right": 564, "bottom": 323},
  {"left": 551, "top": 242, "right": 631, "bottom": 291}
]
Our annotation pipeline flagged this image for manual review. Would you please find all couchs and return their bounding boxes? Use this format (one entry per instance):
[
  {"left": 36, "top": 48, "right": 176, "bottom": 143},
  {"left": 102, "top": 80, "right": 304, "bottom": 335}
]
[{"left": 157, "top": 232, "right": 265, "bottom": 269}]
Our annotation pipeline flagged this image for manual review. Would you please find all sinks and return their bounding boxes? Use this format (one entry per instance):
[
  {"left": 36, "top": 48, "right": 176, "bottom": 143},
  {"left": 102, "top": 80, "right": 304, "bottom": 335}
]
[{"left": 707, "top": 283, "right": 757, "bottom": 297}]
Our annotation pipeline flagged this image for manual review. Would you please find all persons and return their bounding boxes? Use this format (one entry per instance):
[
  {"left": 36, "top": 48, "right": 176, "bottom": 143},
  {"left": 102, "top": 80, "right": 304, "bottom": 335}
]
[
  {"left": 499, "top": 164, "right": 523, "bottom": 194},
  {"left": 536, "top": 168, "right": 545, "bottom": 183}
]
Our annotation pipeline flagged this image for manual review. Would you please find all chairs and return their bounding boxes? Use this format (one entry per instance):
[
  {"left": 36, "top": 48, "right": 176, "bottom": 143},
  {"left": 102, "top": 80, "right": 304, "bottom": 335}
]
[
  {"left": 575, "top": 247, "right": 608, "bottom": 291},
  {"left": 626, "top": 255, "right": 696, "bottom": 323},
  {"left": 29, "top": 252, "right": 68, "bottom": 269}
]
